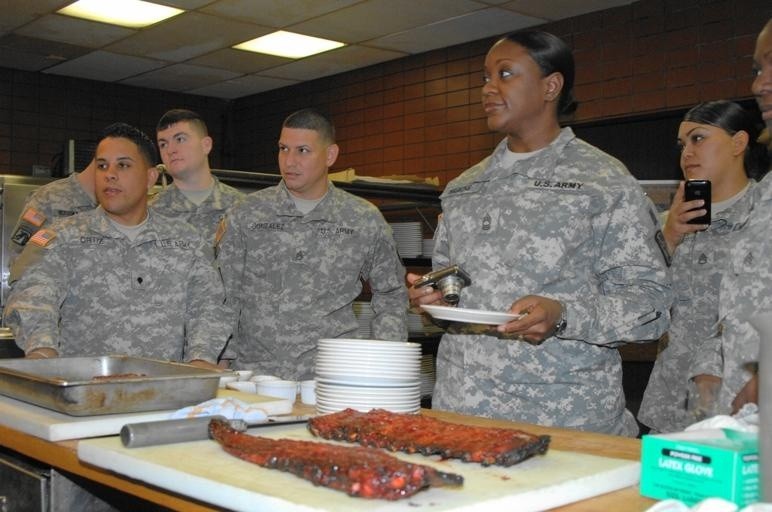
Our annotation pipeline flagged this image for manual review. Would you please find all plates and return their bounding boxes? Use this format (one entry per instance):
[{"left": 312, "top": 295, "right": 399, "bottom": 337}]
[
  {"left": 421, "top": 303, "right": 522, "bottom": 326},
  {"left": 311, "top": 335, "right": 423, "bottom": 418}
]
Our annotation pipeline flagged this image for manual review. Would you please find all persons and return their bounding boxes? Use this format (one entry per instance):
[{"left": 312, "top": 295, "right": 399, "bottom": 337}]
[
  {"left": 1, "top": 109, "right": 410, "bottom": 381},
  {"left": 407, "top": 31, "right": 674, "bottom": 438},
  {"left": 638, "top": 101, "right": 759, "bottom": 436},
  {"left": 687, "top": 15, "right": 772, "bottom": 431}
]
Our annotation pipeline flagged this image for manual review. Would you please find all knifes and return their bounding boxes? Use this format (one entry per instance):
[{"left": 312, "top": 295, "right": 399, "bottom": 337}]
[{"left": 121, "top": 414, "right": 316, "bottom": 448}]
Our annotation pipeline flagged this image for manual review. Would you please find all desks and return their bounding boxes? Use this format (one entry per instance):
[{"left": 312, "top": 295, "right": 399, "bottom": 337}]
[{"left": 0, "top": 388, "right": 662, "bottom": 512}]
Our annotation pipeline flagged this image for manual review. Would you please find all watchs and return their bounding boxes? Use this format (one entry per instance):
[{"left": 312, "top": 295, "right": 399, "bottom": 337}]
[{"left": 554, "top": 300, "right": 566, "bottom": 337}]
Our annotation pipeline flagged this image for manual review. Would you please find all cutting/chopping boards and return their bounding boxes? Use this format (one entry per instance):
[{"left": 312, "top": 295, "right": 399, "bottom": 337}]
[
  {"left": 75, "top": 419, "right": 645, "bottom": 510},
  {"left": 0, "top": 395, "right": 294, "bottom": 442}
]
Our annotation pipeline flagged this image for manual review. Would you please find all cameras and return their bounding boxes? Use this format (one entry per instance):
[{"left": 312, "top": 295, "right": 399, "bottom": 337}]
[{"left": 414, "top": 265, "right": 472, "bottom": 303}]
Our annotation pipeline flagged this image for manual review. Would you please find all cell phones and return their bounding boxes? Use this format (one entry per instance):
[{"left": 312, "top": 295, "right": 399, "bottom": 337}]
[{"left": 685, "top": 179, "right": 711, "bottom": 225}]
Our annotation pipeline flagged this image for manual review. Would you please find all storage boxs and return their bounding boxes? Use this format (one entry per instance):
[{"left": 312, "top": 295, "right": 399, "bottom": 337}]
[{"left": 639, "top": 425, "right": 757, "bottom": 512}]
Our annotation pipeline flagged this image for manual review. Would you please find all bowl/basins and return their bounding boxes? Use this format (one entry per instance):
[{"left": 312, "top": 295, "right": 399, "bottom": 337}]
[{"left": 219, "top": 369, "right": 316, "bottom": 406}]
